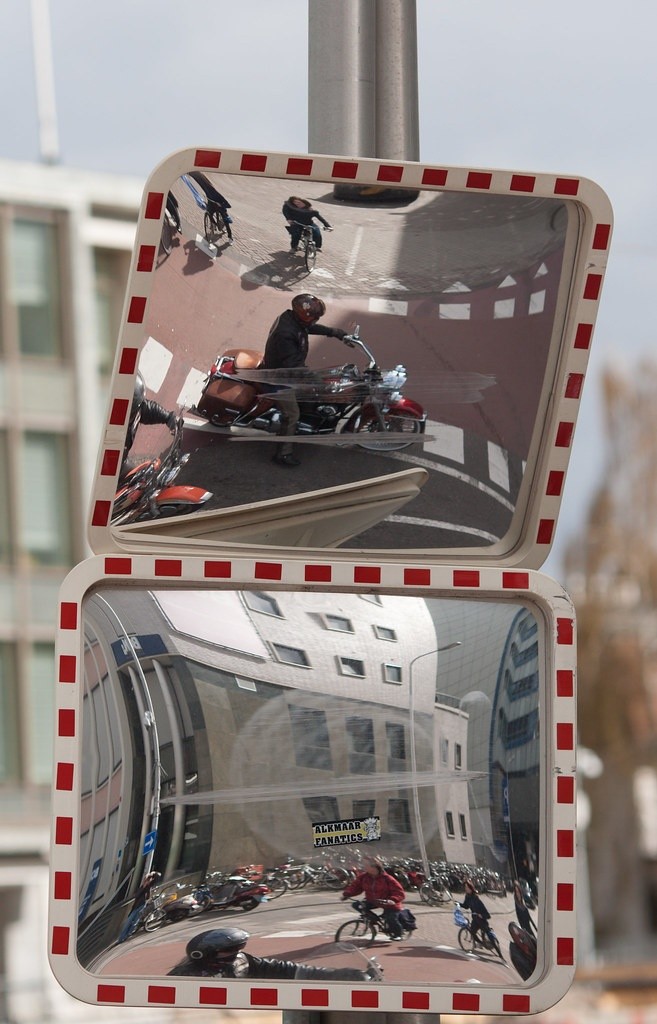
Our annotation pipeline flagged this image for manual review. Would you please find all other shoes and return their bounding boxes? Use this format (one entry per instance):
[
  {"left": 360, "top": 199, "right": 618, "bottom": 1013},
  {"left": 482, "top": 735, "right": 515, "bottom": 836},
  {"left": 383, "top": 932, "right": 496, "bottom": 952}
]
[
  {"left": 229, "top": 238, "right": 234, "bottom": 246},
  {"left": 275, "top": 451, "right": 301, "bottom": 466},
  {"left": 390, "top": 936, "right": 402, "bottom": 942},
  {"left": 290, "top": 247, "right": 296, "bottom": 253}
]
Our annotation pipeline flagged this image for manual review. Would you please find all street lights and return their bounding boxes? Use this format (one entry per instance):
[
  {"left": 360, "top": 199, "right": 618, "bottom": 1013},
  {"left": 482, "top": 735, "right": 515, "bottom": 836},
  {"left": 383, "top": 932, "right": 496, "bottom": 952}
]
[{"left": 409, "top": 640, "right": 463, "bottom": 881}]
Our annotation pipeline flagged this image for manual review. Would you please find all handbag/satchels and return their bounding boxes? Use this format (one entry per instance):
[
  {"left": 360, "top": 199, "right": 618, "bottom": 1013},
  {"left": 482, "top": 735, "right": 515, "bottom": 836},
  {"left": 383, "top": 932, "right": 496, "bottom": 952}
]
[
  {"left": 397, "top": 909, "right": 417, "bottom": 932},
  {"left": 455, "top": 909, "right": 465, "bottom": 927}
]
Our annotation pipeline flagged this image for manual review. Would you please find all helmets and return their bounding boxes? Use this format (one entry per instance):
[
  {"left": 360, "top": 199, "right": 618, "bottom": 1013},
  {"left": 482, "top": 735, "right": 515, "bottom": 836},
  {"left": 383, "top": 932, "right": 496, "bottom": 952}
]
[
  {"left": 292, "top": 294, "right": 326, "bottom": 328},
  {"left": 131, "top": 371, "right": 146, "bottom": 413},
  {"left": 187, "top": 929, "right": 249, "bottom": 968}
]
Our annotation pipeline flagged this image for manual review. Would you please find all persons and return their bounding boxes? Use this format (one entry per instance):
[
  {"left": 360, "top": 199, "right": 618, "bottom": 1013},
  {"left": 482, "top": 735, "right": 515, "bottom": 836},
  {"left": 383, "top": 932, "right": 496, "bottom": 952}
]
[
  {"left": 340, "top": 856, "right": 409, "bottom": 942},
  {"left": 511, "top": 881, "right": 537, "bottom": 943},
  {"left": 259, "top": 292, "right": 359, "bottom": 470},
  {"left": 204, "top": 189, "right": 238, "bottom": 246},
  {"left": 165, "top": 193, "right": 183, "bottom": 235},
  {"left": 282, "top": 197, "right": 334, "bottom": 253},
  {"left": 128, "top": 369, "right": 180, "bottom": 462},
  {"left": 168, "top": 928, "right": 379, "bottom": 981},
  {"left": 455, "top": 878, "right": 495, "bottom": 948},
  {"left": 118, "top": 873, "right": 154, "bottom": 946}
]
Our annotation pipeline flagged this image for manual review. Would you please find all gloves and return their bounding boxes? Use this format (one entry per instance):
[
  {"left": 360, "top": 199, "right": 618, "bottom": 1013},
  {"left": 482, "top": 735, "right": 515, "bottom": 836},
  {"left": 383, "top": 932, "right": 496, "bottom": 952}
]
[
  {"left": 329, "top": 327, "right": 348, "bottom": 342},
  {"left": 165, "top": 413, "right": 185, "bottom": 432}
]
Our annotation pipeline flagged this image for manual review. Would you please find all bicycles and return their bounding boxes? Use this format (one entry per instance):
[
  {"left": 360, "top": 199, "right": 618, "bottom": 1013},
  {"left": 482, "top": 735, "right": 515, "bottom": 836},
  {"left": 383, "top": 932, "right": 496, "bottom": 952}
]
[
  {"left": 159, "top": 208, "right": 184, "bottom": 256},
  {"left": 448, "top": 901, "right": 503, "bottom": 959},
  {"left": 290, "top": 218, "right": 332, "bottom": 276},
  {"left": 124, "top": 843, "right": 539, "bottom": 936},
  {"left": 197, "top": 197, "right": 232, "bottom": 250},
  {"left": 505, "top": 922, "right": 542, "bottom": 962},
  {"left": 334, "top": 894, "right": 415, "bottom": 952}
]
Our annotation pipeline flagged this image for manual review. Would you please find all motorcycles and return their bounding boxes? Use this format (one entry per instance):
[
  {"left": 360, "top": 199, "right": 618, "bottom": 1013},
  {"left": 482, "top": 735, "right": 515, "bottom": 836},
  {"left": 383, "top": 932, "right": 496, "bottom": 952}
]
[
  {"left": 109, "top": 404, "right": 216, "bottom": 529},
  {"left": 189, "top": 314, "right": 430, "bottom": 452}
]
